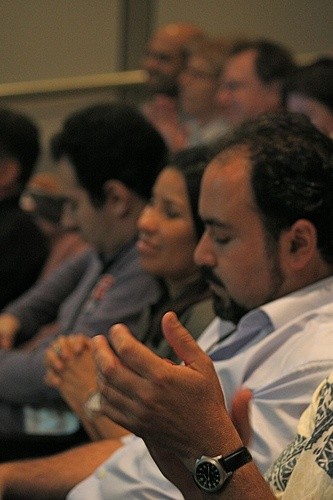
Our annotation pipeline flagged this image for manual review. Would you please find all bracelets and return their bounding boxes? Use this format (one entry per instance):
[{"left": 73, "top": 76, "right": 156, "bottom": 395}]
[{"left": 81, "top": 391, "right": 107, "bottom": 421}]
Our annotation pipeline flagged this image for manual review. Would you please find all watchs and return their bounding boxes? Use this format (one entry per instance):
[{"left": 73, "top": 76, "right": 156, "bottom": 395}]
[{"left": 192, "top": 447, "right": 253, "bottom": 495}]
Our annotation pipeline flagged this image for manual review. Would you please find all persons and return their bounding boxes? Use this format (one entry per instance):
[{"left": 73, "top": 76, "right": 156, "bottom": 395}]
[
  {"left": 0, "top": 21, "right": 333, "bottom": 463},
  {"left": 91, "top": 311, "right": 333, "bottom": 500},
  {"left": 1, "top": 110, "right": 333, "bottom": 500}
]
